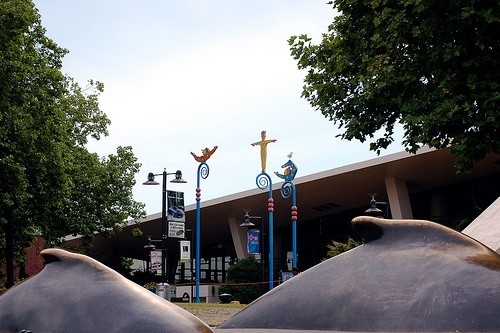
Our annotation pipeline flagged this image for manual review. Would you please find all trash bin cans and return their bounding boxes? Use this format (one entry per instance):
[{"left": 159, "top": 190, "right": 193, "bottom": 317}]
[{"left": 156, "top": 282, "right": 171, "bottom": 302}]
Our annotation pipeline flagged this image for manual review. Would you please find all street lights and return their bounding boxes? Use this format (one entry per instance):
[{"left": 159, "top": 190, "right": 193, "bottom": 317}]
[
  {"left": 364, "top": 192, "right": 389, "bottom": 219},
  {"left": 239, "top": 212, "right": 266, "bottom": 295},
  {"left": 142, "top": 236, "right": 165, "bottom": 283},
  {"left": 141, "top": 167, "right": 189, "bottom": 302}
]
